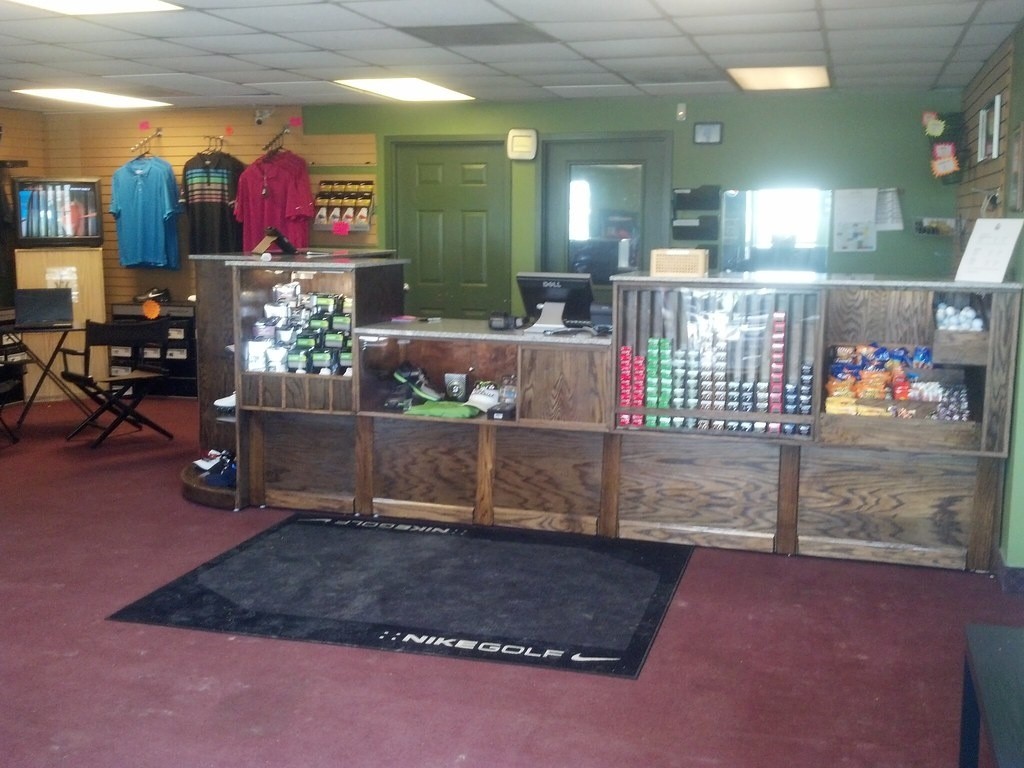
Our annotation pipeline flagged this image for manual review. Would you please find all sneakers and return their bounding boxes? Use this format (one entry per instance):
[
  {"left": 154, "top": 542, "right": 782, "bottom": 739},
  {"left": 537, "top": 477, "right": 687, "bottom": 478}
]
[
  {"left": 133, "top": 288, "right": 170, "bottom": 303},
  {"left": 384, "top": 360, "right": 445, "bottom": 409}
]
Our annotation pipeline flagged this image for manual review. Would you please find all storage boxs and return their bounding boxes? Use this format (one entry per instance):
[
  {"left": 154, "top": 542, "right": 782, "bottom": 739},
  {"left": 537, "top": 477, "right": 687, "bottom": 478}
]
[
  {"left": 649, "top": 249, "right": 708, "bottom": 277},
  {"left": 111, "top": 319, "right": 188, "bottom": 396}
]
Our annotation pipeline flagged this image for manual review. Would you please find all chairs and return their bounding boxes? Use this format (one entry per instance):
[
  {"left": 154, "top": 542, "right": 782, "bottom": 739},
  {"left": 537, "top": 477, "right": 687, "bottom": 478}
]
[
  {"left": 57, "top": 313, "right": 174, "bottom": 448},
  {"left": 0, "top": 332, "right": 35, "bottom": 445}
]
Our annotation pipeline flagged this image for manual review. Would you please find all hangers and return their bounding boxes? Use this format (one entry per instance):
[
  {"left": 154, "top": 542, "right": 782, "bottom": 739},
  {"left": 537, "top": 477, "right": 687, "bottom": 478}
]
[{"left": 129, "top": 134, "right": 290, "bottom": 164}]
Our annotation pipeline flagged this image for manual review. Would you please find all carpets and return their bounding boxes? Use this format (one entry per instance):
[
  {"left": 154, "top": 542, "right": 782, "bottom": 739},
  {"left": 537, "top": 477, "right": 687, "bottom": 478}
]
[{"left": 105, "top": 510, "right": 696, "bottom": 680}]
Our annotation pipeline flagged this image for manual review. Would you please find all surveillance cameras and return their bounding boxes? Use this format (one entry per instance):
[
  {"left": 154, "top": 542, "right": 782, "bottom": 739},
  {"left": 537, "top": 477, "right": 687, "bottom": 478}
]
[{"left": 256, "top": 117, "right": 263, "bottom": 125}]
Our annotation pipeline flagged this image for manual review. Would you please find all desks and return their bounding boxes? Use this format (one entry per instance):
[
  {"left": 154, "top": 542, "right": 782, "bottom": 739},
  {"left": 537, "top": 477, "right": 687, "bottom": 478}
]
[
  {"left": 956, "top": 623, "right": 1023, "bottom": 768},
  {"left": 0, "top": 323, "right": 97, "bottom": 429}
]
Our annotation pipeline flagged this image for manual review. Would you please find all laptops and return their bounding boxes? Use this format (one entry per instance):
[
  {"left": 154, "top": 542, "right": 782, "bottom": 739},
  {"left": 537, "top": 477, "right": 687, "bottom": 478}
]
[{"left": 14, "top": 287, "right": 73, "bottom": 328}]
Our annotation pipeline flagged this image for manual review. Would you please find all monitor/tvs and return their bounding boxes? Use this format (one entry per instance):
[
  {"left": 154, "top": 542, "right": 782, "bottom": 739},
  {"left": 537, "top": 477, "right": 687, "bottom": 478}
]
[
  {"left": 13, "top": 177, "right": 103, "bottom": 248},
  {"left": 516, "top": 273, "right": 594, "bottom": 333}
]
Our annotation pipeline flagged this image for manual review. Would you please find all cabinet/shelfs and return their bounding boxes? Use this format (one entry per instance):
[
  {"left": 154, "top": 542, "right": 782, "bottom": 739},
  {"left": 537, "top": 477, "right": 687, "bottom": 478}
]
[
  {"left": 109, "top": 300, "right": 199, "bottom": 400},
  {"left": 181, "top": 246, "right": 1023, "bottom": 573},
  {"left": 14, "top": 248, "right": 108, "bottom": 403}
]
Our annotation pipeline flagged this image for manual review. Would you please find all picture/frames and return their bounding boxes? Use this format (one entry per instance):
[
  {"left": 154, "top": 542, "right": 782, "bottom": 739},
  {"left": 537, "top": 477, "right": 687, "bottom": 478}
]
[
  {"left": 976, "top": 93, "right": 1000, "bottom": 164},
  {"left": 693, "top": 122, "right": 721, "bottom": 145}
]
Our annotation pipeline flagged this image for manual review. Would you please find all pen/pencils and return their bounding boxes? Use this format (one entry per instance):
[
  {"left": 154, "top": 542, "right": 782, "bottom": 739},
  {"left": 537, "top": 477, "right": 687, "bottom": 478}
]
[{"left": 419, "top": 317, "right": 441, "bottom": 321}]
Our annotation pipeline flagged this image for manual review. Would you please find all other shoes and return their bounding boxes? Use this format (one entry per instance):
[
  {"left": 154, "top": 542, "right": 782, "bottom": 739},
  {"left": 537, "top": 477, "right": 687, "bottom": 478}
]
[{"left": 213, "top": 391, "right": 236, "bottom": 407}]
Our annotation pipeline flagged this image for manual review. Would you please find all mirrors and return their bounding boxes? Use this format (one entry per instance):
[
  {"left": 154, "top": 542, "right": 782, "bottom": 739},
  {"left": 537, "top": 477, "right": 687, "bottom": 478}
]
[
  {"left": 565, "top": 159, "right": 644, "bottom": 285},
  {"left": 719, "top": 187, "right": 833, "bottom": 278}
]
